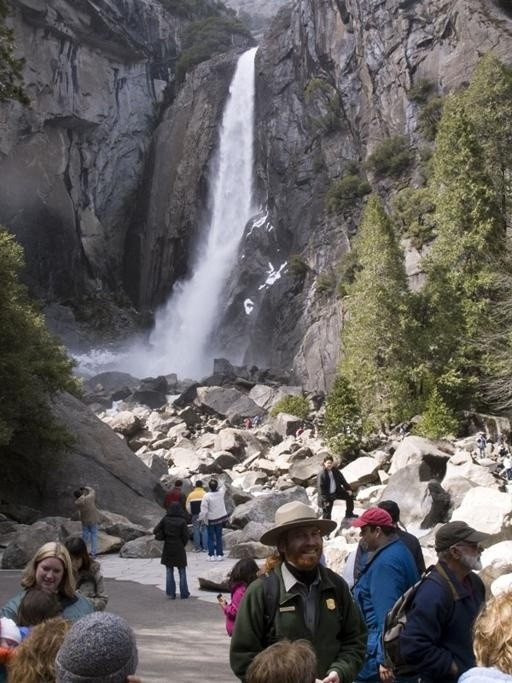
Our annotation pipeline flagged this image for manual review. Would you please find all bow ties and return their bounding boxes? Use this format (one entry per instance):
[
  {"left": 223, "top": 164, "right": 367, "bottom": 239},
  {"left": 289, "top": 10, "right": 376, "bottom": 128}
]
[{"left": 218, "top": 554, "right": 225, "bottom": 561}]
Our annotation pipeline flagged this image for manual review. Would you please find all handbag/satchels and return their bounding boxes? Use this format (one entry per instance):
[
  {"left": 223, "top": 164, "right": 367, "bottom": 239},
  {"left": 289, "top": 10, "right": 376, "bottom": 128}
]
[{"left": 153, "top": 524, "right": 166, "bottom": 541}]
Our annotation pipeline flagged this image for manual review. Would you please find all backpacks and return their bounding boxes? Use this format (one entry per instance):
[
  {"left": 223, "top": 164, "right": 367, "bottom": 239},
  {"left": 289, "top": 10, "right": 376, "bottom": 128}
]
[{"left": 380, "top": 560, "right": 456, "bottom": 678}]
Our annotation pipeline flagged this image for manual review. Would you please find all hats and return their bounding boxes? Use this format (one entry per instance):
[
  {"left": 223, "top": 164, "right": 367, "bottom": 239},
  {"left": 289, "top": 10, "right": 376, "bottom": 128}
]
[
  {"left": 434, "top": 521, "right": 491, "bottom": 552},
  {"left": 259, "top": 501, "right": 338, "bottom": 547},
  {"left": 351, "top": 506, "right": 393, "bottom": 528},
  {"left": 54, "top": 610, "right": 139, "bottom": 683}
]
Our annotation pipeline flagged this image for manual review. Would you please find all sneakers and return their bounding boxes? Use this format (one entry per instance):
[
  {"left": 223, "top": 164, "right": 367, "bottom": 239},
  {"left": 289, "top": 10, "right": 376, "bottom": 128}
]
[
  {"left": 207, "top": 555, "right": 216, "bottom": 561},
  {"left": 345, "top": 512, "right": 359, "bottom": 518}
]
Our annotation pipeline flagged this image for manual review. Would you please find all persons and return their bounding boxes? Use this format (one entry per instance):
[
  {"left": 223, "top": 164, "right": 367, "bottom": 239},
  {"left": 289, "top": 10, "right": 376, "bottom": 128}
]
[
  {"left": 198, "top": 477, "right": 229, "bottom": 561},
  {"left": 164, "top": 478, "right": 186, "bottom": 510},
  {"left": 242, "top": 417, "right": 252, "bottom": 430},
  {"left": 458, "top": 586, "right": 512, "bottom": 681},
  {"left": 229, "top": 501, "right": 369, "bottom": 680},
  {"left": 0, "top": 534, "right": 141, "bottom": 683},
  {"left": 315, "top": 455, "right": 358, "bottom": 538},
  {"left": 476, "top": 432, "right": 511, "bottom": 482},
  {"left": 246, "top": 638, "right": 317, "bottom": 680},
  {"left": 353, "top": 500, "right": 426, "bottom": 584},
  {"left": 219, "top": 558, "right": 260, "bottom": 636},
  {"left": 397, "top": 521, "right": 493, "bottom": 680},
  {"left": 349, "top": 507, "right": 418, "bottom": 680},
  {"left": 71, "top": 483, "right": 102, "bottom": 556},
  {"left": 251, "top": 414, "right": 261, "bottom": 428},
  {"left": 184, "top": 479, "right": 209, "bottom": 552},
  {"left": 152, "top": 500, "right": 193, "bottom": 600}
]
